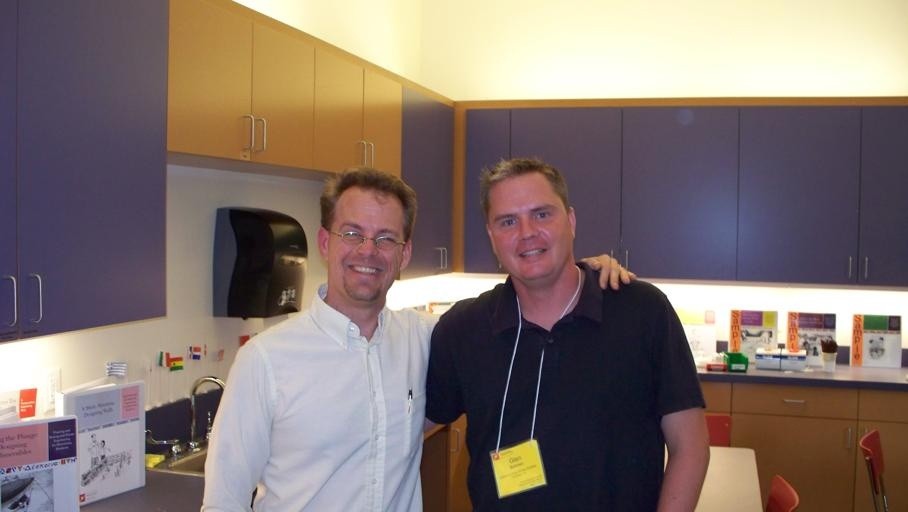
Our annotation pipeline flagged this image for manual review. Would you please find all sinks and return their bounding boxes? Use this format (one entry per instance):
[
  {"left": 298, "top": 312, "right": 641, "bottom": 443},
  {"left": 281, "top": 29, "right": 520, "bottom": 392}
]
[{"left": 157, "top": 452, "right": 204, "bottom": 479}]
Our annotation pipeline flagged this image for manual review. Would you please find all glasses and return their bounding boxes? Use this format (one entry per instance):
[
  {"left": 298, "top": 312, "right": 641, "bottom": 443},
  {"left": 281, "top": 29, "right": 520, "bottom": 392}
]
[{"left": 330, "top": 231, "right": 407, "bottom": 251}]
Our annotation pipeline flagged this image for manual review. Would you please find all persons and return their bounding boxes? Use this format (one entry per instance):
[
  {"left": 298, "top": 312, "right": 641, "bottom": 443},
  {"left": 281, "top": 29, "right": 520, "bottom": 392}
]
[
  {"left": 199, "top": 166, "right": 637, "bottom": 512},
  {"left": 424, "top": 155, "right": 710, "bottom": 512}
]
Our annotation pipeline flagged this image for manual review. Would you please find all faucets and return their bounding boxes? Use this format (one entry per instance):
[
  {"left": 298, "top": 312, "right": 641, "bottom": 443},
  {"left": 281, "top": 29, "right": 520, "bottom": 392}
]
[{"left": 186, "top": 376, "right": 224, "bottom": 446}]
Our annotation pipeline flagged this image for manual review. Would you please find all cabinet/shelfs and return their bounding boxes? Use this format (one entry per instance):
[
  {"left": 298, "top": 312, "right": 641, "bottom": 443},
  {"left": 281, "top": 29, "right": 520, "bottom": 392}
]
[
  {"left": 420, "top": 411, "right": 475, "bottom": 511},
  {"left": 167, "top": 1, "right": 314, "bottom": 172},
  {"left": 2, "top": 1, "right": 169, "bottom": 345},
  {"left": 313, "top": 44, "right": 402, "bottom": 182},
  {"left": 737, "top": 106, "right": 908, "bottom": 287},
  {"left": 464, "top": 109, "right": 510, "bottom": 274},
  {"left": 511, "top": 107, "right": 739, "bottom": 281},
  {"left": 400, "top": 85, "right": 455, "bottom": 281},
  {"left": 698, "top": 376, "right": 908, "bottom": 512}
]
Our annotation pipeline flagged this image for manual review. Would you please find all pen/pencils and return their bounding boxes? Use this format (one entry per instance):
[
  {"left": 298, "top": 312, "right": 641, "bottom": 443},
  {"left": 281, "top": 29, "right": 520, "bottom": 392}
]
[{"left": 408, "top": 388, "right": 412, "bottom": 412}]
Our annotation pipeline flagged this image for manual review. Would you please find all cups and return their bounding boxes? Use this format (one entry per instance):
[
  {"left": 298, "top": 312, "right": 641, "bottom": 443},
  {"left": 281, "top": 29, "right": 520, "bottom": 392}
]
[{"left": 821, "top": 351, "right": 838, "bottom": 373}]
